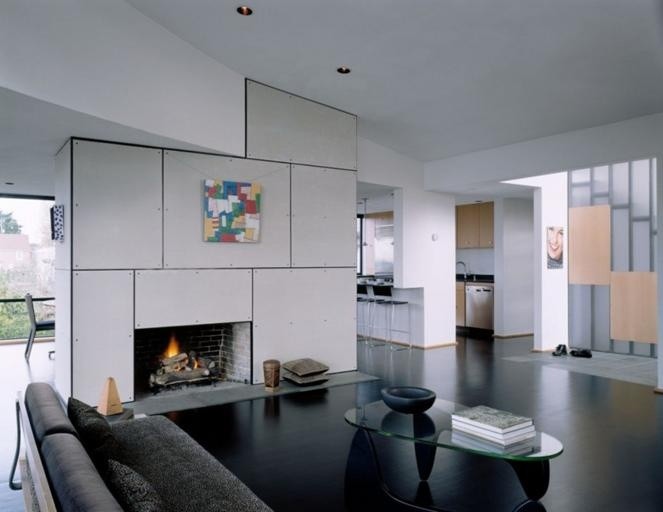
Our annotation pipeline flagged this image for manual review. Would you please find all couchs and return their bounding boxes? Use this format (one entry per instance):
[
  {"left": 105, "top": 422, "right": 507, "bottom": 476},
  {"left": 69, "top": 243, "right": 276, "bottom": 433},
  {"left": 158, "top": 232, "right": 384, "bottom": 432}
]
[{"left": 11, "top": 382, "right": 271, "bottom": 512}]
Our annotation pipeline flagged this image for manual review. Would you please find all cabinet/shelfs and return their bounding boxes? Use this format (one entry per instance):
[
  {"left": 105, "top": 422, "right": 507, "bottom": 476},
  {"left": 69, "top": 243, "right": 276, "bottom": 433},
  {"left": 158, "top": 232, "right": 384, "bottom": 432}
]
[
  {"left": 455, "top": 200, "right": 495, "bottom": 250},
  {"left": 457, "top": 283, "right": 495, "bottom": 340}
]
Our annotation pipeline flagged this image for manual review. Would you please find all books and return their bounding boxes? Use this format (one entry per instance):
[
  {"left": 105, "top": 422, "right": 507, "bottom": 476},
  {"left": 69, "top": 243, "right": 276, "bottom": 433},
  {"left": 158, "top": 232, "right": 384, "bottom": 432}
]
[{"left": 451, "top": 405, "right": 535, "bottom": 448}]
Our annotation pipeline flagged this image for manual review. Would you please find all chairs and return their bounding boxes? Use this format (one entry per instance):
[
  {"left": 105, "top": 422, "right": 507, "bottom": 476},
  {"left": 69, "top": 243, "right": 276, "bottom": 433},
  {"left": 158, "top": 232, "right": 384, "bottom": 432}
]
[
  {"left": 356, "top": 282, "right": 414, "bottom": 355},
  {"left": 25, "top": 292, "right": 55, "bottom": 359}
]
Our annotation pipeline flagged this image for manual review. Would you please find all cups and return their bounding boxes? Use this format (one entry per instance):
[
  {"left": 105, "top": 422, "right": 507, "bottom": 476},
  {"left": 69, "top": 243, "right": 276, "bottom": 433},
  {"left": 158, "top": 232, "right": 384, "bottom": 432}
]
[{"left": 262, "top": 359, "right": 281, "bottom": 393}]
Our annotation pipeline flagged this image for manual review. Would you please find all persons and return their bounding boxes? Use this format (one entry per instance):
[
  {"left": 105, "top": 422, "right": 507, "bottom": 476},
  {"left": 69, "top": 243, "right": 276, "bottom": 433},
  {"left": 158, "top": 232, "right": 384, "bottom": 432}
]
[{"left": 547, "top": 226, "right": 563, "bottom": 269}]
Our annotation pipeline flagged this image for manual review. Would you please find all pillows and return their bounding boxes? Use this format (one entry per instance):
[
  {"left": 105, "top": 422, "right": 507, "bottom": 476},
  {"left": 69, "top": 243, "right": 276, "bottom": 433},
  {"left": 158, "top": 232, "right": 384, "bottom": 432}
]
[
  {"left": 107, "top": 457, "right": 164, "bottom": 512},
  {"left": 67, "top": 395, "right": 113, "bottom": 453}
]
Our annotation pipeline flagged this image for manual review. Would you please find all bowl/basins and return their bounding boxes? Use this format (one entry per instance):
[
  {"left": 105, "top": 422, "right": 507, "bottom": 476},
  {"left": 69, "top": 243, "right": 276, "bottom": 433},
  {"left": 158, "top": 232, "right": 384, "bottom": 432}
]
[
  {"left": 378, "top": 385, "right": 437, "bottom": 415},
  {"left": 381, "top": 409, "right": 438, "bottom": 439}
]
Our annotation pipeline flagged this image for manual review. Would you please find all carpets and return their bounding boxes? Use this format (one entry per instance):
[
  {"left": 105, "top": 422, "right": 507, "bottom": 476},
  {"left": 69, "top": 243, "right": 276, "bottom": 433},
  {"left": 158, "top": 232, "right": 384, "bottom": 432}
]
[{"left": 120, "top": 369, "right": 380, "bottom": 422}]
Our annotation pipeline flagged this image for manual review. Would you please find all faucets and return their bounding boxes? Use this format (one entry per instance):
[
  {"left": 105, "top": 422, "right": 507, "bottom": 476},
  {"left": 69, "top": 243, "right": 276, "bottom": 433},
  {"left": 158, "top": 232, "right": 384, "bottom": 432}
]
[{"left": 456, "top": 261, "right": 467, "bottom": 277}]
